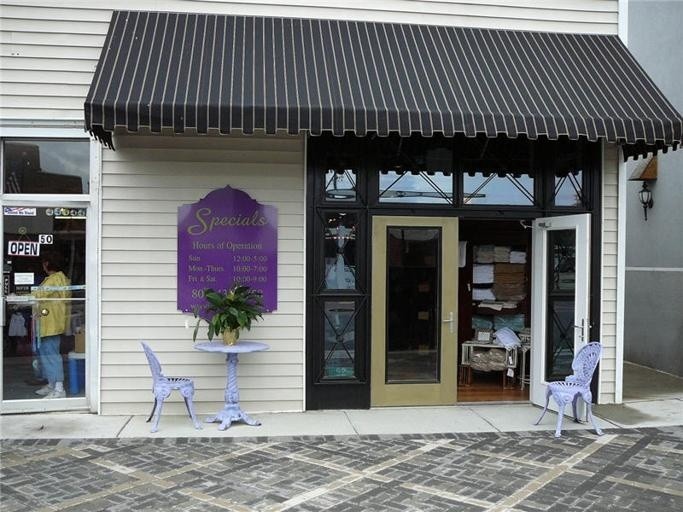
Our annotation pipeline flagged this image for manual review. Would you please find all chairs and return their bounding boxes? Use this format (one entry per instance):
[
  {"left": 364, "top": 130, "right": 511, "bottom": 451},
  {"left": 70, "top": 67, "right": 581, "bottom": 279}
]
[
  {"left": 140, "top": 341, "right": 203, "bottom": 432},
  {"left": 535, "top": 342, "right": 604, "bottom": 437}
]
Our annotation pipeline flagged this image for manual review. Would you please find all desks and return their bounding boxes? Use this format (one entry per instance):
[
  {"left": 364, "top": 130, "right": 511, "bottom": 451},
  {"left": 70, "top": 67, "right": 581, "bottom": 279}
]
[
  {"left": 193, "top": 342, "right": 268, "bottom": 431},
  {"left": 68, "top": 349, "right": 85, "bottom": 394},
  {"left": 462, "top": 334, "right": 530, "bottom": 391}
]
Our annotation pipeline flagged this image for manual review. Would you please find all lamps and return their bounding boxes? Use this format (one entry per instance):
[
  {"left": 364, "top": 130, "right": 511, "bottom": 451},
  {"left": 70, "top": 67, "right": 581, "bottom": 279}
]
[{"left": 639, "top": 181, "right": 653, "bottom": 221}]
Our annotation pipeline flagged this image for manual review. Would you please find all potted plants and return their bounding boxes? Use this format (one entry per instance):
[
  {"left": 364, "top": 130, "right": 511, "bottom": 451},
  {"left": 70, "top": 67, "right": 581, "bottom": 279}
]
[{"left": 193, "top": 281, "right": 266, "bottom": 345}]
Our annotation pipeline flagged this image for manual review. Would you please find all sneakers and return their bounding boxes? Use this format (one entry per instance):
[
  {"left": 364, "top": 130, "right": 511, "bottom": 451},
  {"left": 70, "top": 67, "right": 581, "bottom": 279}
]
[
  {"left": 44, "top": 390, "right": 64, "bottom": 400},
  {"left": 36, "top": 382, "right": 54, "bottom": 395}
]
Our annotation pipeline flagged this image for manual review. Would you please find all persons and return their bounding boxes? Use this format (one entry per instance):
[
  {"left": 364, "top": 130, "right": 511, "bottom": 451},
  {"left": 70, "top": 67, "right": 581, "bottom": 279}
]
[{"left": 8, "top": 255, "right": 72, "bottom": 399}]
